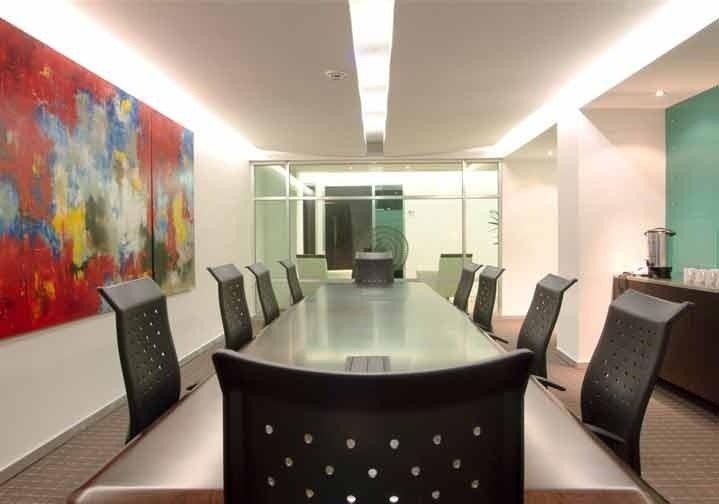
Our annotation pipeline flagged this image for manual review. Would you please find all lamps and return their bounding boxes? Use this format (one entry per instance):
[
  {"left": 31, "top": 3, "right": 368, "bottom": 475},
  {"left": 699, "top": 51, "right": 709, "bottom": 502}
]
[
  {"left": 347, "top": 0, "right": 399, "bottom": 155},
  {"left": 655, "top": 88, "right": 665, "bottom": 96}
]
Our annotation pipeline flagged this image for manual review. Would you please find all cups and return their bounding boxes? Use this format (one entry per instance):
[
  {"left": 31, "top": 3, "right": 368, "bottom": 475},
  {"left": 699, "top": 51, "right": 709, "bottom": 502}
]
[{"left": 684, "top": 267, "right": 719, "bottom": 290}]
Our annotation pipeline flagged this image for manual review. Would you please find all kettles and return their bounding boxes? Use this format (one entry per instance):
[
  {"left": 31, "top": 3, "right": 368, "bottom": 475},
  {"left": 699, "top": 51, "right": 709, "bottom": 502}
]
[{"left": 644, "top": 227, "right": 676, "bottom": 278}]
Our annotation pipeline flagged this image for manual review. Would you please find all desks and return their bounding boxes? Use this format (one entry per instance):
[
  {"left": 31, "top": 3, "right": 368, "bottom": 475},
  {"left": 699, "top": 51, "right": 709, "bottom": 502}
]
[
  {"left": 611, "top": 274, "right": 718, "bottom": 407},
  {"left": 65, "top": 279, "right": 669, "bottom": 504}
]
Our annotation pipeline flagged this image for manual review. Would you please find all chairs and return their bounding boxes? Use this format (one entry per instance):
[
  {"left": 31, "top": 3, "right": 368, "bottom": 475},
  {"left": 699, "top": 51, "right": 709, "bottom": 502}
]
[
  {"left": 279, "top": 259, "right": 303, "bottom": 302},
  {"left": 473, "top": 264, "right": 509, "bottom": 344},
  {"left": 96, "top": 276, "right": 181, "bottom": 443},
  {"left": 516, "top": 273, "right": 578, "bottom": 391},
  {"left": 417, "top": 254, "right": 473, "bottom": 298},
  {"left": 207, "top": 263, "right": 253, "bottom": 349},
  {"left": 296, "top": 255, "right": 327, "bottom": 278},
  {"left": 453, "top": 261, "right": 483, "bottom": 311},
  {"left": 580, "top": 287, "right": 696, "bottom": 477},
  {"left": 352, "top": 250, "right": 394, "bottom": 281},
  {"left": 209, "top": 348, "right": 535, "bottom": 502},
  {"left": 244, "top": 261, "right": 281, "bottom": 324}
]
